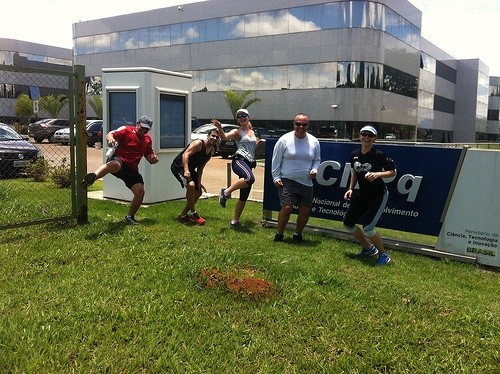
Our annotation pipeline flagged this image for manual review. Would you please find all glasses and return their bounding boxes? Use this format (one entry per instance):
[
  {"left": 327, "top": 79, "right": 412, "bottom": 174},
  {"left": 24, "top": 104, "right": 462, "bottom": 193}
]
[
  {"left": 237, "top": 113, "right": 248, "bottom": 118},
  {"left": 294, "top": 123, "right": 308, "bottom": 127},
  {"left": 360, "top": 133, "right": 374, "bottom": 138}
]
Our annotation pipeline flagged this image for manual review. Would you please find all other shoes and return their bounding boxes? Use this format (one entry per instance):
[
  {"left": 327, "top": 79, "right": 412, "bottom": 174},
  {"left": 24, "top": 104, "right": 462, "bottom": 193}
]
[
  {"left": 293, "top": 234, "right": 304, "bottom": 242},
  {"left": 274, "top": 233, "right": 284, "bottom": 242}
]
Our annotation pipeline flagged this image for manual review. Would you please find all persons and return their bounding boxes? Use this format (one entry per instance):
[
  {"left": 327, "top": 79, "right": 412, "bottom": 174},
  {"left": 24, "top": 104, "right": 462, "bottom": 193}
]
[
  {"left": 272, "top": 113, "right": 321, "bottom": 243},
  {"left": 170, "top": 129, "right": 219, "bottom": 226},
  {"left": 81, "top": 115, "right": 158, "bottom": 224},
  {"left": 343, "top": 125, "right": 397, "bottom": 266},
  {"left": 211, "top": 109, "right": 266, "bottom": 228}
]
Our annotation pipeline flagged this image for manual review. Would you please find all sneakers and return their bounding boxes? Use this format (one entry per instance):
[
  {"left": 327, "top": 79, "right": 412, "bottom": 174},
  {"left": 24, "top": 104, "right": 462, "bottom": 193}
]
[
  {"left": 178, "top": 215, "right": 194, "bottom": 223},
  {"left": 81, "top": 173, "right": 96, "bottom": 189},
  {"left": 357, "top": 245, "right": 378, "bottom": 258},
  {"left": 220, "top": 188, "right": 227, "bottom": 208},
  {"left": 230, "top": 221, "right": 245, "bottom": 231},
  {"left": 191, "top": 212, "right": 206, "bottom": 224},
  {"left": 123, "top": 216, "right": 137, "bottom": 225},
  {"left": 375, "top": 252, "right": 391, "bottom": 265}
]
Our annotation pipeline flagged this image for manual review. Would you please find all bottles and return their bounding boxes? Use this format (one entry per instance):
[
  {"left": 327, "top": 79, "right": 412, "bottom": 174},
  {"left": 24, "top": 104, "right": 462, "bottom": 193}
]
[{"left": 106, "top": 141, "right": 118, "bottom": 157}]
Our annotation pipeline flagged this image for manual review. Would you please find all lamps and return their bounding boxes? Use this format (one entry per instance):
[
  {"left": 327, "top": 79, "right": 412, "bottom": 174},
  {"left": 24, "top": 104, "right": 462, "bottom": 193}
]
[{"left": 331, "top": 105, "right": 338, "bottom": 110}]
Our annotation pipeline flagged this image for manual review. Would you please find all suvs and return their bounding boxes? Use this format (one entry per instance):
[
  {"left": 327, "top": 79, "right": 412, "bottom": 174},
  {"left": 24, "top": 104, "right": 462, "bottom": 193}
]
[
  {"left": 28, "top": 118, "right": 103, "bottom": 147},
  {"left": 0, "top": 123, "right": 44, "bottom": 178}
]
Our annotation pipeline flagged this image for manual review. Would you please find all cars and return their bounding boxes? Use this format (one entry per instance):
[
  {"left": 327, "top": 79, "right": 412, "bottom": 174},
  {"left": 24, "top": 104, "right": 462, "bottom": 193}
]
[
  {"left": 385, "top": 134, "right": 396, "bottom": 139},
  {"left": 191, "top": 124, "right": 293, "bottom": 159},
  {"left": 320, "top": 126, "right": 338, "bottom": 138}
]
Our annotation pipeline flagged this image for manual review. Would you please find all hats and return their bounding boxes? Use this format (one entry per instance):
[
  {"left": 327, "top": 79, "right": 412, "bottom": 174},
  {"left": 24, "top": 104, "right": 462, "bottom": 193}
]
[
  {"left": 360, "top": 125, "right": 378, "bottom": 136},
  {"left": 237, "top": 108, "right": 249, "bottom": 116},
  {"left": 139, "top": 115, "right": 153, "bottom": 130}
]
